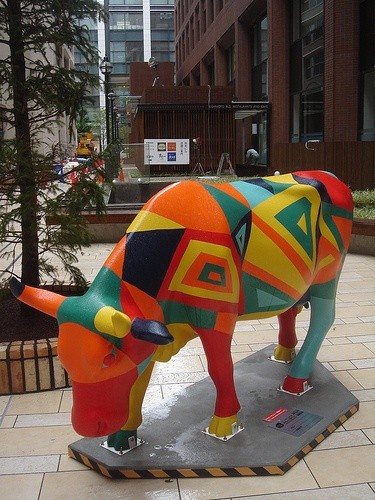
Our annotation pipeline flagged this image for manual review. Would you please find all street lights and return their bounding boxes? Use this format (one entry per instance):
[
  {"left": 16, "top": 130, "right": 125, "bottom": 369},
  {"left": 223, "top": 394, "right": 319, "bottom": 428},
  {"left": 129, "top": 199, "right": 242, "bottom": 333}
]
[
  {"left": 99, "top": 54, "right": 114, "bottom": 146},
  {"left": 107, "top": 89, "right": 120, "bottom": 144}
]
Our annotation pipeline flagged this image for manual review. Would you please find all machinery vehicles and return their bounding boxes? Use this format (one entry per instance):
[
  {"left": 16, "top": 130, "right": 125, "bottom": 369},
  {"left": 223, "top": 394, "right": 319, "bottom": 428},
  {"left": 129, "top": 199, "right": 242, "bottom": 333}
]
[{"left": 74, "top": 130, "right": 95, "bottom": 159}]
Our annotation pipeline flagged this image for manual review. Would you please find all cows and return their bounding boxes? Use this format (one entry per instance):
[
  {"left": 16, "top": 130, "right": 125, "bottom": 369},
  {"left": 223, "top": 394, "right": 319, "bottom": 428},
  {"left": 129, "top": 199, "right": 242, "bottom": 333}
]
[{"left": 9, "top": 171, "right": 354, "bottom": 452}]
